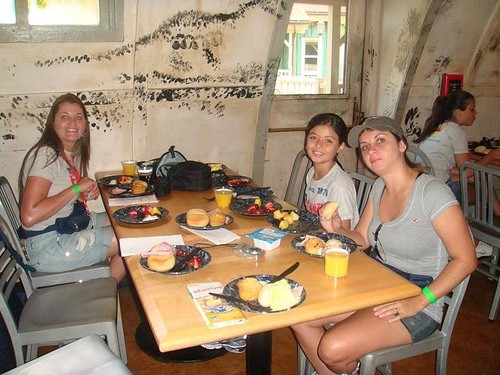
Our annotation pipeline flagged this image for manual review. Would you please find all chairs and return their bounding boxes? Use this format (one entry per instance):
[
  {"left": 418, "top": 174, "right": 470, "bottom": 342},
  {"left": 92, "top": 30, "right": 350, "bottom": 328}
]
[
  {"left": 298, "top": 170, "right": 376, "bottom": 375},
  {"left": 310, "top": 224, "right": 477, "bottom": 375},
  {"left": 285, "top": 149, "right": 312, "bottom": 211},
  {"left": 407, "top": 146, "right": 435, "bottom": 177},
  {"left": 0, "top": 215, "right": 127, "bottom": 367},
  {"left": 0, "top": 333, "right": 134, "bottom": 375},
  {"left": 459, "top": 162, "right": 500, "bottom": 322},
  {"left": 0, "top": 176, "right": 111, "bottom": 290}
]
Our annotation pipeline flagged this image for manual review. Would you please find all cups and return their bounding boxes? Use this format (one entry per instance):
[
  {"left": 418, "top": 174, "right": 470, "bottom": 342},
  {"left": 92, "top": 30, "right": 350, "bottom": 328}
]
[
  {"left": 121, "top": 159, "right": 137, "bottom": 175},
  {"left": 213, "top": 185, "right": 235, "bottom": 208},
  {"left": 137, "top": 166, "right": 153, "bottom": 180},
  {"left": 322, "top": 245, "right": 352, "bottom": 277}
]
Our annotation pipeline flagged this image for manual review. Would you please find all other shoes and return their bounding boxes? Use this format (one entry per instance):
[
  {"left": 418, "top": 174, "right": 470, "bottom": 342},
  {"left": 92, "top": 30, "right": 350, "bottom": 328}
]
[{"left": 475, "top": 241, "right": 493, "bottom": 259}]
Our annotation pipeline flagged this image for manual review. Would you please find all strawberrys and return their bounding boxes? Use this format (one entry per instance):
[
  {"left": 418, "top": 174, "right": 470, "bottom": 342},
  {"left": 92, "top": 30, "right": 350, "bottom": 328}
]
[
  {"left": 187, "top": 256, "right": 202, "bottom": 269},
  {"left": 227, "top": 178, "right": 249, "bottom": 185},
  {"left": 128, "top": 206, "right": 152, "bottom": 217},
  {"left": 247, "top": 203, "right": 276, "bottom": 214}
]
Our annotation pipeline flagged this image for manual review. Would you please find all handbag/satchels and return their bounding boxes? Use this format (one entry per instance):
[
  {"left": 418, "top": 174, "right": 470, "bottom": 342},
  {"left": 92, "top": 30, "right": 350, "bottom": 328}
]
[
  {"left": 168, "top": 161, "right": 213, "bottom": 190},
  {"left": 153, "top": 145, "right": 186, "bottom": 182}
]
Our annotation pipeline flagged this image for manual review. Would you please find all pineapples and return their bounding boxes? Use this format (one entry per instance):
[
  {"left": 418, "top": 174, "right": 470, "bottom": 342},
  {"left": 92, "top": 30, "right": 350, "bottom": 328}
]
[{"left": 272, "top": 210, "right": 300, "bottom": 229}]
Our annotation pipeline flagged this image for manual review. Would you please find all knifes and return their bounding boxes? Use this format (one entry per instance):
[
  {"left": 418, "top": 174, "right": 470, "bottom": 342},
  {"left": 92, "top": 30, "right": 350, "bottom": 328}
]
[{"left": 173, "top": 248, "right": 204, "bottom": 270}]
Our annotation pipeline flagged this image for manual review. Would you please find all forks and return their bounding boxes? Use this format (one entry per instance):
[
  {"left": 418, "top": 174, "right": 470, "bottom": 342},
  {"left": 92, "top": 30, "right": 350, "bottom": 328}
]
[{"left": 208, "top": 291, "right": 272, "bottom": 312}]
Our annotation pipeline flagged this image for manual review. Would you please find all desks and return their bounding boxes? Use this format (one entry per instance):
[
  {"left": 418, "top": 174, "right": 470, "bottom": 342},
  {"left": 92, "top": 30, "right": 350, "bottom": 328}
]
[{"left": 95, "top": 163, "right": 421, "bottom": 375}]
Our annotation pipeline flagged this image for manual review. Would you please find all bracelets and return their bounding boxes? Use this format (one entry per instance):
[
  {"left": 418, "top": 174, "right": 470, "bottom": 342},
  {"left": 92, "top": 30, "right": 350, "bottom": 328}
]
[
  {"left": 70, "top": 185, "right": 81, "bottom": 194},
  {"left": 423, "top": 286, "right": 437, "bottom": 304}
]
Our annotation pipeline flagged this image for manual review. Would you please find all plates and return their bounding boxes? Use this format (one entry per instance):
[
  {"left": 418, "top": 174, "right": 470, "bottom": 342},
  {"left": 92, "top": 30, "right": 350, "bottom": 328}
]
[
  {"left": 174, "top": 210, "right": 233, "bottom": 232},
  {"left": 141, "top": 245, "right": 212, "bottom": 275},
  {"left": 207, "top": 162, "right": 227, "bottom": 172},
  {"left": 223, "top": 274, "right": 306, "bottom": 315},
  {"left": 467, "top": 140, "right": 500, "bottom": 156},
  {"left": 213, "top": 175, "right": 320, "bottom": 230},
  {"left": 113, "top": 204, "right": 169, "bottom": 224},
  {"left": 291, "top": 232, "right": 357, "bottom": 259},
  {"left": 98, "top": 176, "right": 157, "bottom": 197}
]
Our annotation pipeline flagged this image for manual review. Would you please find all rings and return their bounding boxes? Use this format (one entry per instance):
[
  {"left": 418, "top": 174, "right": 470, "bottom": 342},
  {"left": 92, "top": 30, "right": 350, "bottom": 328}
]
[{"left": 393, "top": 308, "right": 397, "bottom": 314}]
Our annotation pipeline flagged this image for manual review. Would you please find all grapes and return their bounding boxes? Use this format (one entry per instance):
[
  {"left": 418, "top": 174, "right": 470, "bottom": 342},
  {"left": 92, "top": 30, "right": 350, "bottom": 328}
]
[{"left": 177, "top": 248, "right": 190, "bottom": 256}]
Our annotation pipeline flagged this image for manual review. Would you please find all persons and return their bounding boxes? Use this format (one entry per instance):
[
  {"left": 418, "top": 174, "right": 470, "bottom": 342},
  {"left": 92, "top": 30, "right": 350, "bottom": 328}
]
[
  {"left": 202, "top": 113, "right": 478, "bottom": 375},
  {"left": 413, "top": 91, "right": 500, "bottom": 217},
  {"left": 17, "top": 92, "right": 128, "bottom": 285}
]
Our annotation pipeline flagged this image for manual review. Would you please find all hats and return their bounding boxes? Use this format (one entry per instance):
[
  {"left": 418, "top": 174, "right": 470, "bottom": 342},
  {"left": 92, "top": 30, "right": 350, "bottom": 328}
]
[{"left": 347, "top": 116, "right": 403, "bottom": 148}]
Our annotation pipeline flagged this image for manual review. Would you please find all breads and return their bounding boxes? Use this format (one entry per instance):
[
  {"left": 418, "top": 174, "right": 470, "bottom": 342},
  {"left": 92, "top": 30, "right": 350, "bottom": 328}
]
[
  {"left": 147, "top": 243, "right": 175, "bottom": 271},
  {"left": 132, "top": 180, "right": 148, "bottom": 194},
  {"left": 474, "top": 145, "right": 492, "bottom": 154},
  {"left": 304, "top": 237, "right": 343, "bottom": 255},
  {"left": 186, "top": 208, "right": 227, "bottom": 227},
  {"left": 237, "top": 276, "right": 297, "bottom": 311},
  {"left": 321, "top": 203, "right": 339, "bottom": 219}
]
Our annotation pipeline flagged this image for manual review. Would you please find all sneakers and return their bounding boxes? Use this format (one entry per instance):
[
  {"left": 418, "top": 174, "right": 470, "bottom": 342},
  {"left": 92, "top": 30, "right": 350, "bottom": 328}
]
[{"left": 202, "top": 336, "right": 248, "bottom": 354}]
